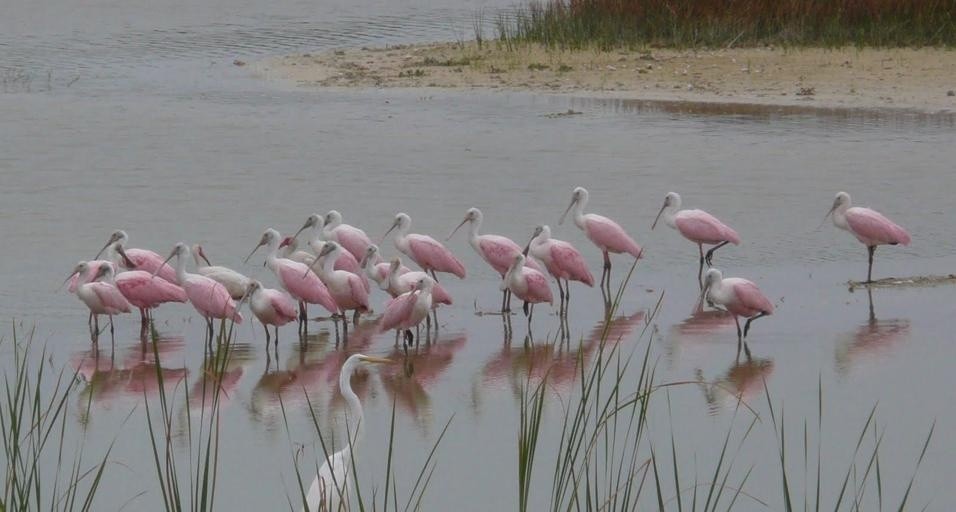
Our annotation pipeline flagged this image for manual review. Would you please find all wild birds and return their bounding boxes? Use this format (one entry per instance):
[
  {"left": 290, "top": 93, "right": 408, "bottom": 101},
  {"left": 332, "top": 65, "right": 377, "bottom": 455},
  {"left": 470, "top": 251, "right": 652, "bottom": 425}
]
[
  {"left": 153, "top": 243, "right": 252, "bottom": 343},
  {"left": 62, "top": 229, "right": 187, "bottom": 355},
  {"left": 821, "top": 189, "right": 915, "bottom": 286},
  {"left": 520, "top": 224, "right": 594, "bottom": 316},
  {"left": 361, "top": 245, "right": 453, "bottom": 355},
  {"left": 301, "top": 352, "right": 395, "bottom": 512},
  {"left": 650, "top": 188, "right": 745, "bottom": 280},
  {"left": 500, "top": 251, "right": 553, "bottom": 322},
  {"left": 558, "top": 187, "right": 645, "bottom": 285},
  {"left": 245, "top": 209, "right": 382, "bottom": 350},
  {"left": 447, "top": 207, "right": 542, "bottom": 313},
  {"left": 237, "top": 279, "right": 297, "bottom": 342},
  {"left": 697, "top": 267, "right": 777, "bottom": 341},
  {"left": 382, "top": 214, "right": 465, "bottom": 283}
]
